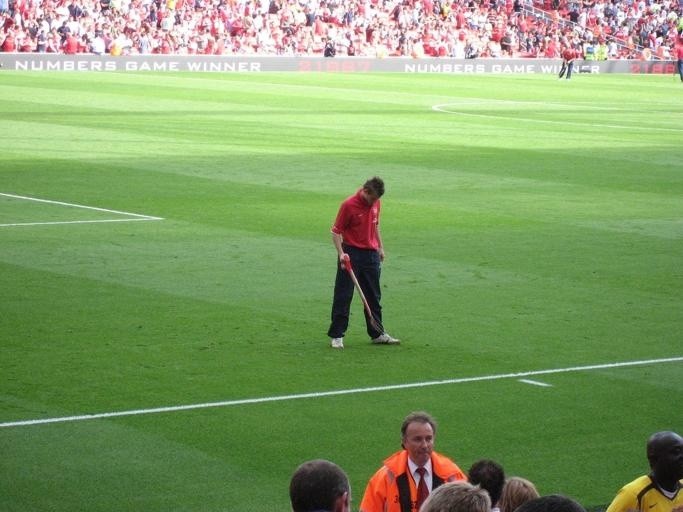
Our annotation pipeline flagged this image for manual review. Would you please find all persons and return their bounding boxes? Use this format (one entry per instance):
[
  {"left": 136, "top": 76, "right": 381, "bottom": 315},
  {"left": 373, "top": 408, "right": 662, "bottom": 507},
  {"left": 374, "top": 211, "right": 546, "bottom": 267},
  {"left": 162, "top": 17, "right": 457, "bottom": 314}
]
[
  {"left": 327, "top": 174, "right": 401, "bottom": 349},
  {"left": 467, "top": 458, "right": 505, "bottom": 512},
  {"left": 606, "top": 430, "right": 682, "bottom": 512},
  {"left": 511, "top": 494, "right": 588, "bottom": 511},
  {"left": 287, "top": 457, "right": 352, "bottom": 511},
  {"left": 358, "top": 411, "right": 467, "bottom": 512},
  {"left": 497, "top": 476, "right": 542, "bottom": 511},
  {"left": 418, "top": 480, "right": 492, "bottom": 512}
]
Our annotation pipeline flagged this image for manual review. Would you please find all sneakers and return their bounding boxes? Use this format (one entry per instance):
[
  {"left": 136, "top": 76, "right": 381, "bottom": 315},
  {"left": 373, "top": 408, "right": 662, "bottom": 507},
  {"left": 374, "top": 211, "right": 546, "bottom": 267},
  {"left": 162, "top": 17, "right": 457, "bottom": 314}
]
[
  {"left": 330, "top": 335, "right": 344, "bottom": 348},
  {"left": 371, "top": 334, "right": 400, "bottom": 345}
]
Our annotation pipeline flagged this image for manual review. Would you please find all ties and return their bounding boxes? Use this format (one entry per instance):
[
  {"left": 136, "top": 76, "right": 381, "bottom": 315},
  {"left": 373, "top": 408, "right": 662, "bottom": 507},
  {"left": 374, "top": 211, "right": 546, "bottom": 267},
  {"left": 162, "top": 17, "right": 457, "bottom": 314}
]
[{"left": 415, "top": 467, "right": 431, "bottom": 512}]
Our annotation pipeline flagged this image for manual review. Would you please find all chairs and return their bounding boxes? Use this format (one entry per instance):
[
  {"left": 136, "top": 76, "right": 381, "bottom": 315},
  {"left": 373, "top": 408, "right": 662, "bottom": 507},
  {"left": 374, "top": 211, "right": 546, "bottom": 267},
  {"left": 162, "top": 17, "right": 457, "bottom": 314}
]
[{"left": 452, "top": 0, "right": 678, "bottom": 61}]
